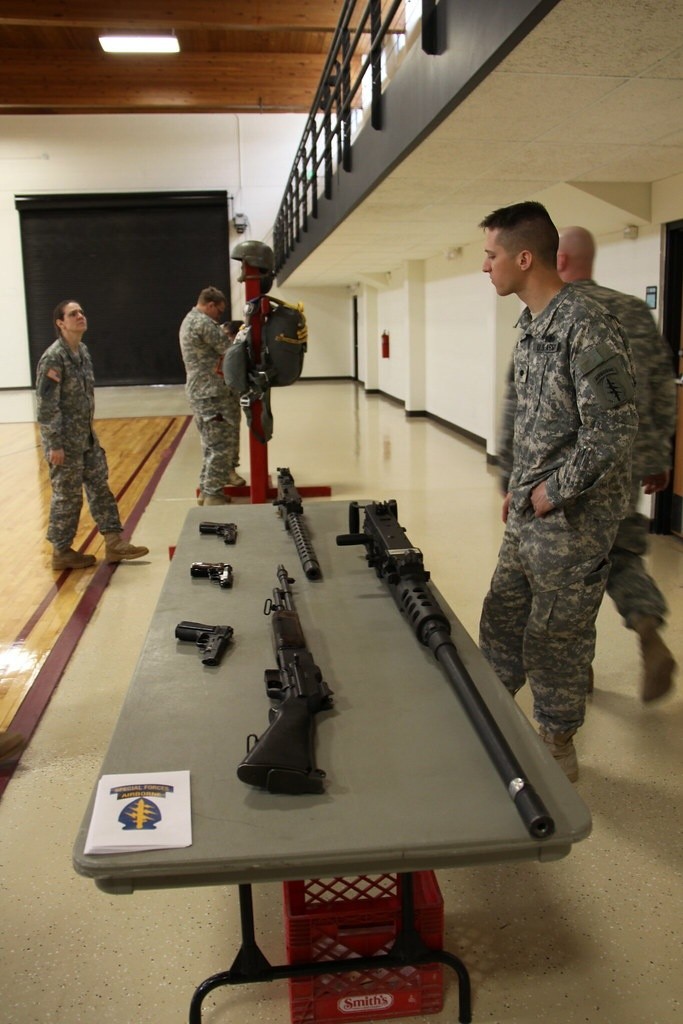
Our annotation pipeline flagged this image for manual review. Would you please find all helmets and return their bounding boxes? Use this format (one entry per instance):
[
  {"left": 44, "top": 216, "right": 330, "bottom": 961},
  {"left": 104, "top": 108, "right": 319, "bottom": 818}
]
[{"left": 231, "top": 241, "right": 275, "bottom": 282}]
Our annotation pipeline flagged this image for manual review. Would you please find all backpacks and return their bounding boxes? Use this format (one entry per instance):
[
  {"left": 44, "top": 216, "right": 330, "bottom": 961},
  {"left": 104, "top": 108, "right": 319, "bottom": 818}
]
[{"left": 240, "top": 294, "right": 308, "bottom": 388}]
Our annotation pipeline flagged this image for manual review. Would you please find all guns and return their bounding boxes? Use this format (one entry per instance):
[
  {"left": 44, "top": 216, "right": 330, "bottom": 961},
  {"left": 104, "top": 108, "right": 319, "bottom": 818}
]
[
  {"left": 198, "top": 521, "right": 239, "bottom": 545},
  {"left": 234, "top": 562, "right": 337, "bottom": 797},
  {"left": 189, "top": 560, "right": 234, "bottom": 590},
  {"left": 175, "top": 620, "right": 235, "bottom": 668}
]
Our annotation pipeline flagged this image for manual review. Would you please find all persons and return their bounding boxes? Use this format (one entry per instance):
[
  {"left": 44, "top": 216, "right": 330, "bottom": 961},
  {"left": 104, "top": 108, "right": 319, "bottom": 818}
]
[
  {"left": 35, "top": 299, "right": 150, "bottom": 568},
  {"left": 180, "top": 285, "right": 249, "bottom": 506},
  {"left": 495, "top": 222, "right": 680, "bottom": 707},
  {"left": 473, "top": 201, "right": 641, "bottom": 783}
]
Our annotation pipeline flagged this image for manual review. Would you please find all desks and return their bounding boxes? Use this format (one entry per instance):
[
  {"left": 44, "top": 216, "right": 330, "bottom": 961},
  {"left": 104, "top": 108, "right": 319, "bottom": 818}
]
[{"left": 72, "top": 499, "right": 592, "bottom": 1024}]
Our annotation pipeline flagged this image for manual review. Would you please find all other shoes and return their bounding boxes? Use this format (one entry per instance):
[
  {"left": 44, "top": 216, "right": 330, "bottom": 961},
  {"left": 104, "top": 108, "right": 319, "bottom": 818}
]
[
  {"left": 539, "top": 726, "right": 579, "bottom": 783},
  {"left": 639, "top": 627, "right": 675, "bottom": 702}
]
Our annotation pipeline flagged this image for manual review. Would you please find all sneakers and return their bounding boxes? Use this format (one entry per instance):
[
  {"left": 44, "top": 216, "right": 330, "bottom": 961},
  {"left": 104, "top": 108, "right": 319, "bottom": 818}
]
[
  {"left": 197, "top": 490, "right": 232, "bottom": 505},
  {"left": 203, "top": 496, "right": 230, "bottom": 506},
  {"left": 52, "top": 545, "right": 96, "bottom": 570},
  {"left": 226, "top": 461, "right": 246, "bottom": 486},
  {"left": 104, "top": 532, "right": 149, "bottom": 562}
]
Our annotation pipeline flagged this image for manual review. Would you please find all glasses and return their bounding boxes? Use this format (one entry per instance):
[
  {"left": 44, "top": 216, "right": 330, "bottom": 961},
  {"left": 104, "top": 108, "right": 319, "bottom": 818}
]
[{"left": 216, "top": 306, "right": 223, "bottom": 316}]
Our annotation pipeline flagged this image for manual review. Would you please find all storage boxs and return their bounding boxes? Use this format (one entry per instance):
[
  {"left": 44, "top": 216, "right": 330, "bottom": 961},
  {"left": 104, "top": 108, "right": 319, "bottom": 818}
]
[{"left": 283, "top": 870, "right": 444, "bottom": 1024}]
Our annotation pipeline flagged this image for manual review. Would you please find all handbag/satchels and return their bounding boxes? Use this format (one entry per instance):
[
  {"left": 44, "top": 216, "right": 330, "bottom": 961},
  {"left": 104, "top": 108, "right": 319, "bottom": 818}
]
[{"left": 223, "top": 342, "right": 274, "bottom": 445}]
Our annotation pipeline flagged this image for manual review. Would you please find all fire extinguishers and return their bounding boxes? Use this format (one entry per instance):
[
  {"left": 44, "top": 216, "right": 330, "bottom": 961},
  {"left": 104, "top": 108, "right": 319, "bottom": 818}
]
[{"left": 381, "top": 330, "right": 390, "bottom": 358}]
